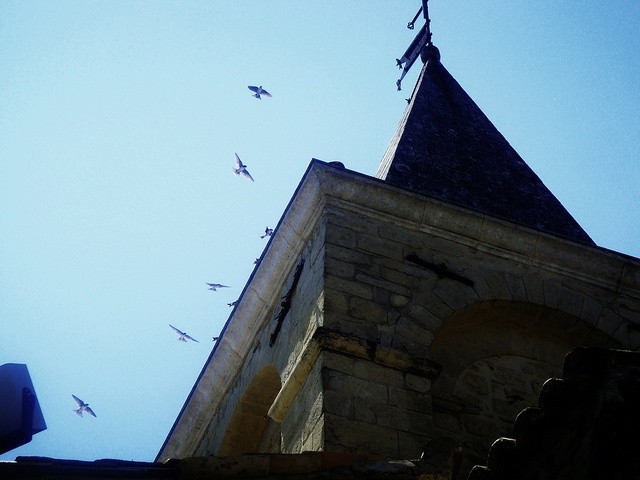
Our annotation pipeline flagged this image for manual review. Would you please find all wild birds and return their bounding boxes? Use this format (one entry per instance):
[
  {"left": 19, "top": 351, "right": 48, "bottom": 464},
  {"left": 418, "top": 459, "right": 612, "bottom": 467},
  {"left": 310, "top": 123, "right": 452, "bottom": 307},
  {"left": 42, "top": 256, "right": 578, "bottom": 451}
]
[
  {"left": 248, "top": 84, "right": 272, "bottom": 101},
  {"left": 71, "top": 394, "right": 97, "bottom": 418},
  {"left": 232, "top": 151, "right": 254, "bottom": 182},
  {"left": 169, "top": 324, "right": 200, "bottom": 343},
  {"left": 259, "top": 225, "right": 273, "bottom": 239},
  {"left": 205, "top": 281, "right": 230, "bottom": 292}
]
